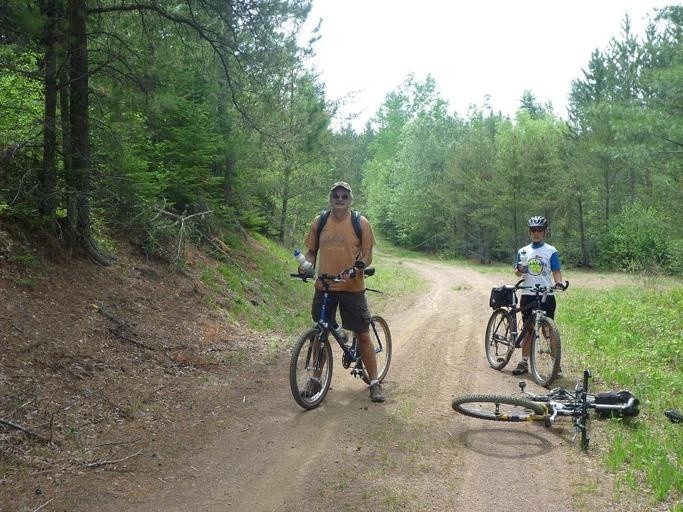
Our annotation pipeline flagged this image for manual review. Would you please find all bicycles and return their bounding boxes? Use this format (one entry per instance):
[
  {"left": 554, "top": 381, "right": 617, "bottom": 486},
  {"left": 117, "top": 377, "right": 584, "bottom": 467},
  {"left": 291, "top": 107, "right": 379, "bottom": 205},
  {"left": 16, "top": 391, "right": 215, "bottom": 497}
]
[
  {"left": 447, "top": 371, "right": 642, "bottom": 455},
  {"left": 284, "top": 263, "right": 396, "bottom": 413},
  {"left": 477, "top": 278, "right": 575, "bottom": 389}
]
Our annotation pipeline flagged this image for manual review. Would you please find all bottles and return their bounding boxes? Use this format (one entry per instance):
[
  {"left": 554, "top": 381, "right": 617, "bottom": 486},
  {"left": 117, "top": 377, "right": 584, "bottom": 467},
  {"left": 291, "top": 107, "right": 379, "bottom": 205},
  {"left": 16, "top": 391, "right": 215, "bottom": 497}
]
[
  {"left": 520, "top": 249, "right": 529, "bottom": 274},
  {"left": 294, "top": 249, "right": 314, "bottom": 276}
]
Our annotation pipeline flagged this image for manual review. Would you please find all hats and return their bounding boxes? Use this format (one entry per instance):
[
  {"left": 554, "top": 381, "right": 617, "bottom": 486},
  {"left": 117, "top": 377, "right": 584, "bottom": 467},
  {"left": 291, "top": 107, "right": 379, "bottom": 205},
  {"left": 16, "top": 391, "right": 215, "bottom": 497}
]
[{"left": 331, "top": 181, "right": 351, "bottom": 192}]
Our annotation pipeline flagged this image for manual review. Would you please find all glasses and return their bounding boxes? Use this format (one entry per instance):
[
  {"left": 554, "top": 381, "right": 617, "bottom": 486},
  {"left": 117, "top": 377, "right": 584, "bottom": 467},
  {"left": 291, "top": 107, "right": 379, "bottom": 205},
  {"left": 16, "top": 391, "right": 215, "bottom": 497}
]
[
  {"left": 530, "top": 229, "right": 544, "bottom": 232},
  {"left": 333, "top": 194, "right": 348, "bottom": 199}
]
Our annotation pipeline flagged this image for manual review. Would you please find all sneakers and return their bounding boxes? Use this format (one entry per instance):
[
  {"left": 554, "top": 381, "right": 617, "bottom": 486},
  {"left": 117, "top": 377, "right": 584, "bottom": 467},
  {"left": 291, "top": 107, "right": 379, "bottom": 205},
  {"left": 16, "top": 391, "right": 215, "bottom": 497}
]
[
  {"left": 513, "top": 362, "right": 528, "bottom": 375},
  {"left": 300, "top": 379, "right": 320, "bottom": 397},
  {"left": 370, "top": 384, "right": 385, "bottom": 402}
]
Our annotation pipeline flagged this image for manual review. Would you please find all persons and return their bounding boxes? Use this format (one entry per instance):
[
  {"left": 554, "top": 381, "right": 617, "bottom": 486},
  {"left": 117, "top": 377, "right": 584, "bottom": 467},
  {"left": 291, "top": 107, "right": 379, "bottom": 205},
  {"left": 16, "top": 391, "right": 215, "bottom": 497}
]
[
  {"left": 511, "top": 215, "right": 562, "bottom": 378},
  {"left": 297, "top": 181, "right": 385, "bottom": 401}
]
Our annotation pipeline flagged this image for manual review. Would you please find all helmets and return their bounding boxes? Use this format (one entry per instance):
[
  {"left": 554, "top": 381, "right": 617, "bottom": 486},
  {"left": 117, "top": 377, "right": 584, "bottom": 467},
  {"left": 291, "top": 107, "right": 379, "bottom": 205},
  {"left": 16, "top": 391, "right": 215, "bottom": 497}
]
[{"left": 527, "top": 216, "right": 548, "bottom": 228}]
